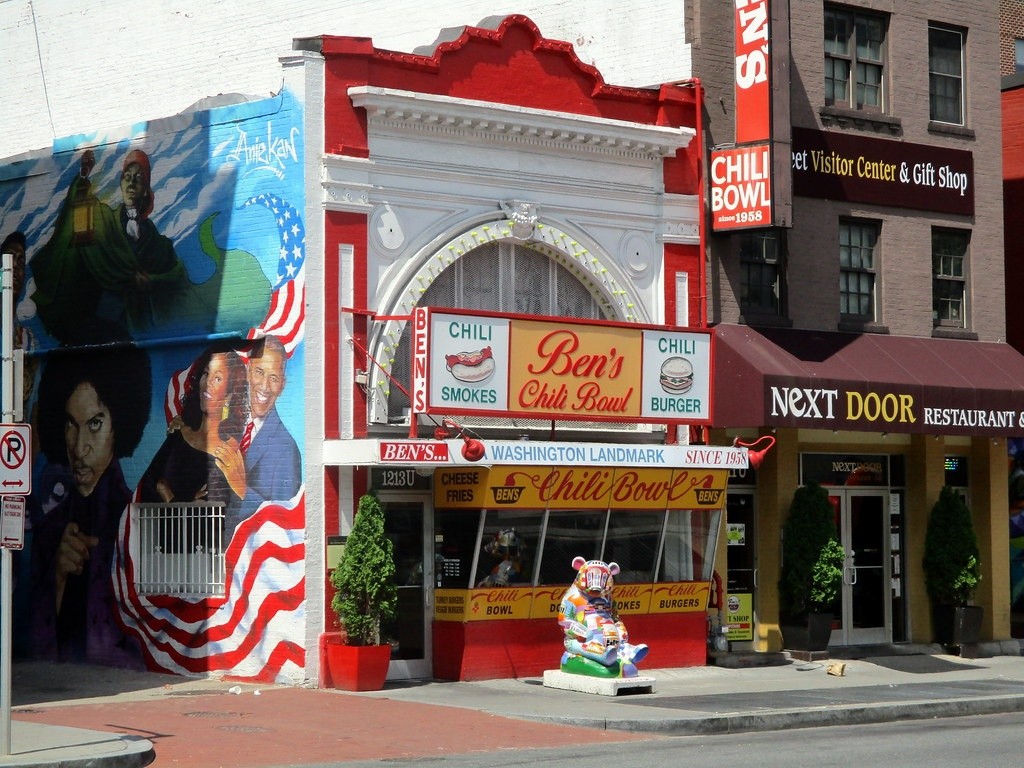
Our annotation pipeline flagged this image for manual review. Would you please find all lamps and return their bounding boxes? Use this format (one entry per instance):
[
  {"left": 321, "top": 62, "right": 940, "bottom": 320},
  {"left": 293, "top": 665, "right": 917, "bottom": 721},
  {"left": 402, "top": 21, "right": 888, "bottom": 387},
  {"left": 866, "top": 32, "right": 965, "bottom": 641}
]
[
  {"left": 733, "top": 436, "right": 776, "bottom": 472},
  {"left": 435, "top": 420, "right": 486, "bottom": 462}
]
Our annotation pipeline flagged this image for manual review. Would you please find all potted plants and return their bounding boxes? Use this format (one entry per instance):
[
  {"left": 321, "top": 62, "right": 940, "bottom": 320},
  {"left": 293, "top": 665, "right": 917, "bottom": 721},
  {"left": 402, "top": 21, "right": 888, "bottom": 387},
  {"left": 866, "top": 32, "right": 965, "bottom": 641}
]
[
  {"left": 922, "top": 488, "right": 986, "bottom": 643},
  {"left": 328, "top": 490, "right": 396, "bottom": 691},
  {"left": 778, "top": 482, "right": 848, "bottom": 650}
]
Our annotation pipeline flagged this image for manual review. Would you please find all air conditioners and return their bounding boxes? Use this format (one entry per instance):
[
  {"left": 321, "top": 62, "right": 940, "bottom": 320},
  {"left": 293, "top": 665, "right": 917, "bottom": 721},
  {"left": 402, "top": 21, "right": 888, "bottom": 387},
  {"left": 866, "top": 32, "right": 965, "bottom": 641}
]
[{"left": 743, "top": 237, "right": 779, "bottom": 267}]
